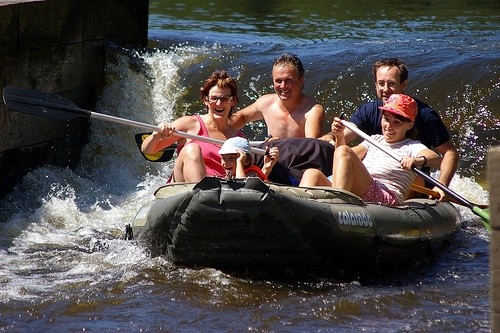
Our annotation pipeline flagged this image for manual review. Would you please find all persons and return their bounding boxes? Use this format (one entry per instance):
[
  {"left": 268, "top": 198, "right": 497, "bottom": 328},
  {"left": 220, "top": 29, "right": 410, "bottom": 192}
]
[
  {"left": 227, "top": 53, "right": 324, "bottom": 140},
  {"left": 141, "top": 71, "right": 278, "bottom": 184},
  {"left": 218, "top": 136, "right": 268, "bottom": 182},
  {"left": 298, "top": 94, "right": 442, "bottom": 205},
  {"left": 317, "top": 57, "right": 458, "bottom": 201}
]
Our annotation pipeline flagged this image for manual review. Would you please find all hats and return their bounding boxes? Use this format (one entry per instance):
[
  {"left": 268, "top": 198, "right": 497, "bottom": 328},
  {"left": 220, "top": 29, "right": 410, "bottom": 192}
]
[
  {"left": 219, "top": 136, "right": 250, "bottom": 155},
  {"left": 378, "top": 94, "right": 418, "bottom": 122}
]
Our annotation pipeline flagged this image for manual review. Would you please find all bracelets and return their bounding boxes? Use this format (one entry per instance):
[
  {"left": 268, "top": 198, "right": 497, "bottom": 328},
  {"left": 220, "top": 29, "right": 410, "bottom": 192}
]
[{"left": 419, "top": 155, "right": 427, "bottom": 168}]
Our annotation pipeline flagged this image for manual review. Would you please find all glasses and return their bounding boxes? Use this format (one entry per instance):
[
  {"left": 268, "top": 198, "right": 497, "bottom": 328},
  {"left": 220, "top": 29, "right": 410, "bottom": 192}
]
[{"left": 206, "top": 96, "right": 233, "bottom": 103}]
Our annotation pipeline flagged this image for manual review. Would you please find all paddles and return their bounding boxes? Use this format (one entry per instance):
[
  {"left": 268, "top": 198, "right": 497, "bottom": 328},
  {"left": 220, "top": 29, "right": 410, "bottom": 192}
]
[
  {"left": 3, "top": 86, "right": 274, "bottom": 158},
  {"left": 340, "top": 120, "right": 491, "bottom": 235}
]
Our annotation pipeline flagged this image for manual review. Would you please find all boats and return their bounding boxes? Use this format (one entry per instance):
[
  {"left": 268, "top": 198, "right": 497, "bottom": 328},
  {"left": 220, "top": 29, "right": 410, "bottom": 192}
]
[{"left": 145, "top": 176, "right": 461, "bottom": 281}]
